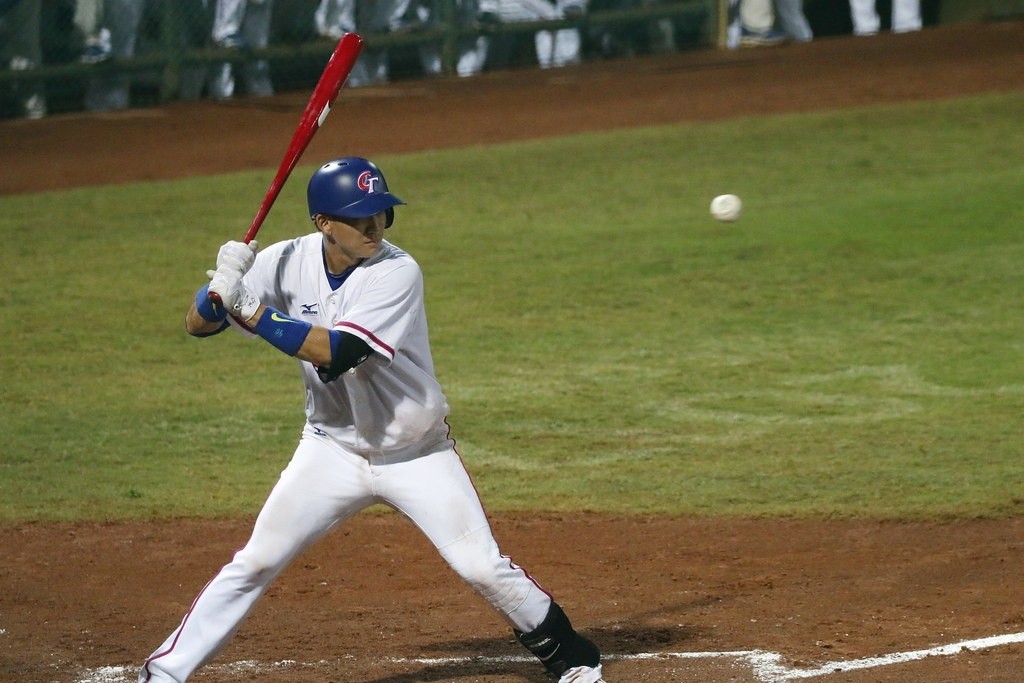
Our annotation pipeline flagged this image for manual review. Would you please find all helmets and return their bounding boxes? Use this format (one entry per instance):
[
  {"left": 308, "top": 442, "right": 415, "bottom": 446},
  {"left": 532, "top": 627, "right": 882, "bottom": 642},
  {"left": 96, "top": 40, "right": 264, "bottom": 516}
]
[{"left": 307, "top": 158, "right": 408, "bottom": 229}]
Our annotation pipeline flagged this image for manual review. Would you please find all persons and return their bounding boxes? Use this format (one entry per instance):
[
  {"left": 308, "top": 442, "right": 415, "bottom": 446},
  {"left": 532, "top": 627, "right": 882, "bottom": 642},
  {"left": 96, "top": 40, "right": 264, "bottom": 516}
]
[
  {"left": 0, "top": 0, "right": 921, "bottom": 121},
  {"left": 137, "top": 158, "right": 607, "bottom": 683}
]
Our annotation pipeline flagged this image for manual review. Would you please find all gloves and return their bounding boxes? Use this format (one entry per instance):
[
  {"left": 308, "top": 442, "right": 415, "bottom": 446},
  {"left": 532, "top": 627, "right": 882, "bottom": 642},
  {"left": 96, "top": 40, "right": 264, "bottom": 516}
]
[
  {"left": 206, "top": 263, "right": 261, "bottom": 324},
  {"left": 216, "top": 239, "right": 258, "bottom": 281}
]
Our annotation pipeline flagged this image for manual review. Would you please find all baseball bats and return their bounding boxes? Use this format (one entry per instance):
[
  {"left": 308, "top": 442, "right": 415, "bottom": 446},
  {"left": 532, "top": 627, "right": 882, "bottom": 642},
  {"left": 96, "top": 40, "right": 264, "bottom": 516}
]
[{"left": 208, "top": 31, "right": 364, "bottom": 301}]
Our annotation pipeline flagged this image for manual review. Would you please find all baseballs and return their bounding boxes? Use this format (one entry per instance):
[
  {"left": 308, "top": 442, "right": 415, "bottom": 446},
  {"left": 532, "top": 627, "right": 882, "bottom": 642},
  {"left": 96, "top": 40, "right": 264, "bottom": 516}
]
[{"left": 710, "top": 194, "right": 745, "bottom": 223}]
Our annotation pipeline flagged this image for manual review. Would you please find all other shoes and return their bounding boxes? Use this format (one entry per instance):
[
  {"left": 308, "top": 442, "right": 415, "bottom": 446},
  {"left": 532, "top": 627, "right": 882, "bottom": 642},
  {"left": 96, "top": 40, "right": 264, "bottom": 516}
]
[{"left": 558, "top": 664, "right": 605, "bottom": 683}]
